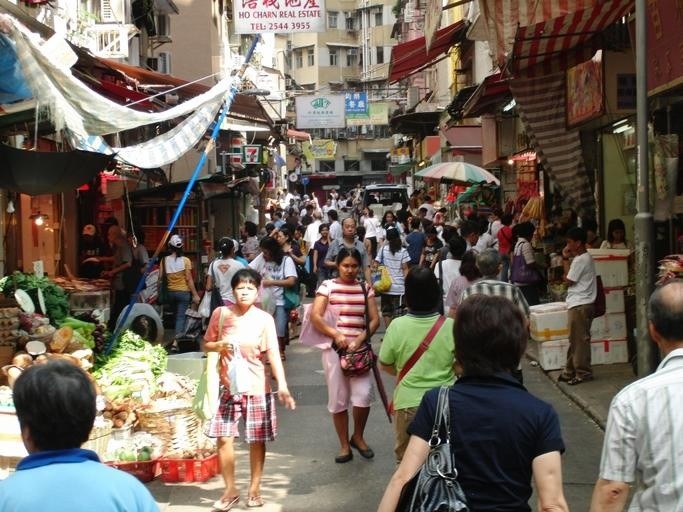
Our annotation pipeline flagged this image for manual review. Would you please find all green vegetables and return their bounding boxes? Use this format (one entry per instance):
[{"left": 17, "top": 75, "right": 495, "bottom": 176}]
[{"left": 92, "top": 350, "right": 155, "bottom": 392}]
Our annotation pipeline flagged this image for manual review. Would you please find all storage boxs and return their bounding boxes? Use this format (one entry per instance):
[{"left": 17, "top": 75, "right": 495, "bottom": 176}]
[
  {"left": 536, "top": 339, "right": 570, "bottom": 371},
  {"left": 603, "top": 289, "right": 624, "bottom": 315},
  {"left": 586, "top": 248, "right": 630, "bottom": 289},
  {"left": 589, "top": 315, "right": 628, "bottom": 342},
  {"left": 591, "top": 342, "right": 629, "bottom": 365},
  {"left": 529, "top": 302, "right": 571, "bottom": 342}
]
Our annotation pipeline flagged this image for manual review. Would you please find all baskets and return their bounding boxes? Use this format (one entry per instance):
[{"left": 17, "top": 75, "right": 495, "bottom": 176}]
[{"left": 83, "top": 406, "right": 220, "bottom": 483}]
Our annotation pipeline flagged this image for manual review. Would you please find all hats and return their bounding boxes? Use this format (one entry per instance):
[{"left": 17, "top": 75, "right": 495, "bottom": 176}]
[{"left": 168, "top": 235, "right": 184, "bottom": 248}]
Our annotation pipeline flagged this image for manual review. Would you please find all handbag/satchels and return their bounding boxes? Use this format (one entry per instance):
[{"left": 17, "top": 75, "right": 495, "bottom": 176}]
[
  {"left": 283, "top": 277, "right": 300, "bottom": 310},
  {"left": 197, "top": 287, "right": 224, "bottom": 325},
  {"left": 158, "top": 275, "right": 168, "bottom": 305},
  {"left": 595, "top": 276, "right": 605, "bottom": 317},
  {"left": 122, "top": 259, "right": 146, "bottom": 292},
  {"left": 340, "top": 342, "right": 374, "bottom": 377},
  {"left": 509, "top": 256, "right": 542, "bottom": 284},
  {"left": 405, "top": 444, "right": 471, "bottom": 511},
  {"left": 192, "top": 352, "right": 221, "bottom": 421},
  {"left": 299, "top": 267, "right": 309, "bottom": 284},
  {"left": 297, "top": 303, "right": 342, "bottom": 350},
  {"left": 370, "top": 266, "right": 392, "bottom": 293}
]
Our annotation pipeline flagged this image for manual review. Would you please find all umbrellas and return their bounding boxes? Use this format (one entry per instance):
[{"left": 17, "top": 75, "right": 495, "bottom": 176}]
[{"left": 413, "top": 161, "right": 501, "bottom": 189}]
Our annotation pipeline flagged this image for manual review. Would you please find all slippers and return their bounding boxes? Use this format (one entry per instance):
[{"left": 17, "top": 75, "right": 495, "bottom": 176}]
[
  {"left": 248, "top": 495, "right": 263, "bottom": 507},
  {"left": 170, "top": 346, "right": 180, "bottom": 352},
  {"left": 212, "top": 495, "right": 240, "bottom": 512}
]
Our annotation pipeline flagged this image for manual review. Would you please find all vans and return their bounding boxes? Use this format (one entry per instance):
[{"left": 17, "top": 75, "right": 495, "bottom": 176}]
[{"left": 362, "top": 184, "right": 408, "bottom": 217}]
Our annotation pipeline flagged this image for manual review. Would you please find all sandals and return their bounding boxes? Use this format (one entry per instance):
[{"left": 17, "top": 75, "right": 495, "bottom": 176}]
[
  {"left": 567, "top": 377, "right": 584, "bottom": 385},
  {"left": 558, "top": 374, "right": 571, "bottom": 381}
]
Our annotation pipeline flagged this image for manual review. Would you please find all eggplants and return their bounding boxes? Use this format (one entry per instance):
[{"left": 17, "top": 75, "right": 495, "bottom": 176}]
[{"left": 74, "top": 310, "right": 104, "bottom": 353}]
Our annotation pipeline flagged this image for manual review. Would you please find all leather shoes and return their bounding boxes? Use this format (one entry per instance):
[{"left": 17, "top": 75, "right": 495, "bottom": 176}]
[
  {"left": 350, "top": 435, "right": 374, "bottom": 458},
  {"left": 336, "top": 450, "right": 353, "bottom": 463}
]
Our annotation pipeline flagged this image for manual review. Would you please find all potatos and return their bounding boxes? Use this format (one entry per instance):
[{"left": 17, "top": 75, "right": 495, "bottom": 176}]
[{"left": 183, "top": 447, "right": 216, "bottom": 460}]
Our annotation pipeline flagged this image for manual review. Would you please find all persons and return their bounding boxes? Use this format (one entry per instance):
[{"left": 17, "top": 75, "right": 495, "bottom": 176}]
[
  {"left": 378, "top": 265, "right": 459, "bottom": 465},
  {"left": 373, "top": 293, "right": 569, "bottom": 511},
  {"left": 587, "top": 277, "right": 683, "bottom": 512},
  {"left": 199, "top": 268, "right": 297, "bottom": 511},
  {"left": 309, "top": 247, "right": 380, "bottom": 463},
  {"left": 558, "top": 227, "right": 598, "bottom": 386},
  {"left": 81, "top": 183, "right": 548, "bottom": 385},
  {"left": 600, "top": 219, "right": 636, "bottom": 310},
  {"left": 0, "top": 359, "right": 162, "bottom": 510}
]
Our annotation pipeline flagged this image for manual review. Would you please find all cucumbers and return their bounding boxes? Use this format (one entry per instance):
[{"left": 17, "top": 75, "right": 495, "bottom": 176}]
[{"left": 58, "top": 316, "right": 96, "bottom": 348}]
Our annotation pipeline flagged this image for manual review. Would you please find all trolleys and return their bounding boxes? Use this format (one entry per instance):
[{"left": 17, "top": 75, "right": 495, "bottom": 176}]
[{"left": 175, "top": 299, "right": 201, "bottom": 349}]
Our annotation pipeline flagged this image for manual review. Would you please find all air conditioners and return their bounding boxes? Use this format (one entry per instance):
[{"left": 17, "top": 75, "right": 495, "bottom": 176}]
[{"left": 101, "top": 0, "right": 121, "bottom": 22}]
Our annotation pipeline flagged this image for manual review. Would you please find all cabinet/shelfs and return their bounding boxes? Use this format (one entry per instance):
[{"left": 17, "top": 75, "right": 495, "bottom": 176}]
[{"left": 134, "top": 204, "right": 200, "bottom": 303}]
